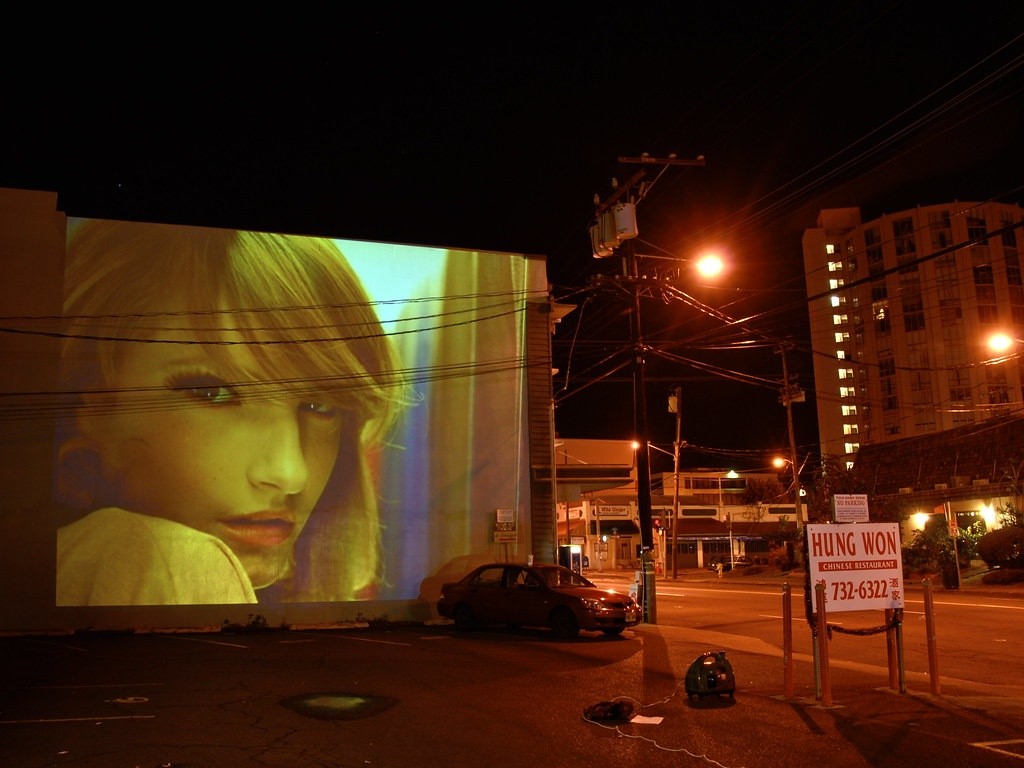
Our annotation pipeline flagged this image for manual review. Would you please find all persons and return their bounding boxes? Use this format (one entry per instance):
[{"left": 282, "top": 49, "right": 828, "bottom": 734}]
[{"left": 57, "top": 221, "right": 412, "bottom": 609}]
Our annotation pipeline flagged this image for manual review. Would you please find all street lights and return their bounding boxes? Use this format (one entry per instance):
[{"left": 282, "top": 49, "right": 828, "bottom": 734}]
[
  {"left": 631, "top": 249, "right": 727, "bottom": 547},
  {"left": 773, "top": 458, "right": 803, "bottom": 544},
  {"left": 630, "top": 437, "right": 680, "bottom": 579}
]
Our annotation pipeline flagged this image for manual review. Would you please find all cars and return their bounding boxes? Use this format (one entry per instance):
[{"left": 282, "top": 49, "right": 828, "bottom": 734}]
[{"left": 436, "top": 560, "right": 643, "bottom": 641}]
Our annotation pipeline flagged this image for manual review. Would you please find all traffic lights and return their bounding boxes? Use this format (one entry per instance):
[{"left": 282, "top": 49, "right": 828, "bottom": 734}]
[
  {"left": 655, "top": 516, "right": 664, "bottom": 536},
  {"left": 726, "top": 514, "right": 732, "bottom": 530}
]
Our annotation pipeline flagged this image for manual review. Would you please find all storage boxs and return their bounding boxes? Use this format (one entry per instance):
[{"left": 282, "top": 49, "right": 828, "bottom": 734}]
[
  {"left": 973, "top": 479, "right": 989, "bottom": 486},
  {"left": 951, "top": 476, "right": 971, "bottom": 487},
  {"left": 935, "top": 484, "right": 948, "bottom": 490},
  {"left": 899, "top": 488, "right": 912, "bottom": 494}
]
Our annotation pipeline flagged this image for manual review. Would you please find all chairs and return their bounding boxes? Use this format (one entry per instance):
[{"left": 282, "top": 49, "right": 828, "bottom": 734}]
[{"left": 521, "top": 573, "right": 538, "bottom": 590}]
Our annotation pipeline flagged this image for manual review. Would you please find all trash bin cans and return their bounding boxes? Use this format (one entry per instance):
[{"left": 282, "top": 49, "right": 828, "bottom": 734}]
[
  {"left": 941, "top": 566, "right": 959, "bottom": 589},
  {"left": 628, "top": 571, "right": 641, "bottom": 603}
]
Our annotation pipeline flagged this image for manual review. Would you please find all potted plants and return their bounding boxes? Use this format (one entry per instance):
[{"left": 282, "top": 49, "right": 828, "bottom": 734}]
[
  {"left": 957, "top": 527, "right": 972, "bottom": 568},
  {"left": 901, "top": 529, "right": 954, "bottom": 583}
]
